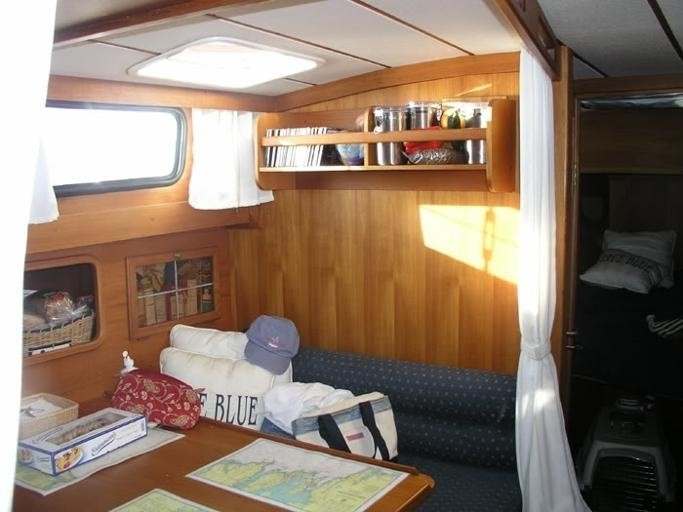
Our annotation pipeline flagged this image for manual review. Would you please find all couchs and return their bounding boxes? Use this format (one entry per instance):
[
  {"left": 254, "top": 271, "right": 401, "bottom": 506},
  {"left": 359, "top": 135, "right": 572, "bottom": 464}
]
[{"left": 288, "top": 348, "right": 524, "bottom": 512}]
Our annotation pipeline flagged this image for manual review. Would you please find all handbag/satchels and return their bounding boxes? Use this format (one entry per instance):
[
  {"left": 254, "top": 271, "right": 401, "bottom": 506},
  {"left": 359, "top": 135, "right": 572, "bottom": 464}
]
[
  {"left": 109, "top": 369, "right": 203, "bottom": 427},
  {"left": 290, "top": 390, "right": 400, "bottom": 464}
]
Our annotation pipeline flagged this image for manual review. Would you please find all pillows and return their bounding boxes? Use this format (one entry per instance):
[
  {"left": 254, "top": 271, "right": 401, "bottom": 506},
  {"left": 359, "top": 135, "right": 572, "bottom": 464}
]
[
  {"left": 169, "top": 323, "right": 249, "bottom": 357},
  {"left": 601, "top": 230, "right": 676, "bottom": 285},
  {"left": 578, "top": 248, "right": 669, "bottom": 295},
  {"left": 158, "top": 349, "right": 289, "bottom": 432}
]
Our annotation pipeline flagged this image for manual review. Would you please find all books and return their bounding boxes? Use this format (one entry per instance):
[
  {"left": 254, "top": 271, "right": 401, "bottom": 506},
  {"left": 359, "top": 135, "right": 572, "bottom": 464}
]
[{"left": 266, "top": 127, "right": 343, "bottom": 166}]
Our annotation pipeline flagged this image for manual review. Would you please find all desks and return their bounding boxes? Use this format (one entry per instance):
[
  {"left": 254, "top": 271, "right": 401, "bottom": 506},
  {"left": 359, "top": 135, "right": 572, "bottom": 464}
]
[{"left": 15, "top": 397, "right": 429, "bottom": 512}]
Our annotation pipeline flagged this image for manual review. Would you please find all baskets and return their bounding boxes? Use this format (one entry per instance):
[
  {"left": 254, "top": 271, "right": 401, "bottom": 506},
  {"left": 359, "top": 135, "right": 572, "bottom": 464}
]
[{"left": 23, "top": 290, "right": 95, "bottom": 355}]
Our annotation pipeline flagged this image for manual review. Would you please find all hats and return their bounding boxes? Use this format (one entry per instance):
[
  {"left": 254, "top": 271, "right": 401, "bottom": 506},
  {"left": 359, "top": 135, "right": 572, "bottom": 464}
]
[{"left": 244, "top": 314, "right": 302, "bottom": 376}]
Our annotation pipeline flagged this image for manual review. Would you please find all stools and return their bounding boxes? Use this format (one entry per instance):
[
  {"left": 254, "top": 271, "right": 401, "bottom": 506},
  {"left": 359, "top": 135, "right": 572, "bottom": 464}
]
[{"left": 574, "top": 404, "right": 676, "bottom": 506}]
